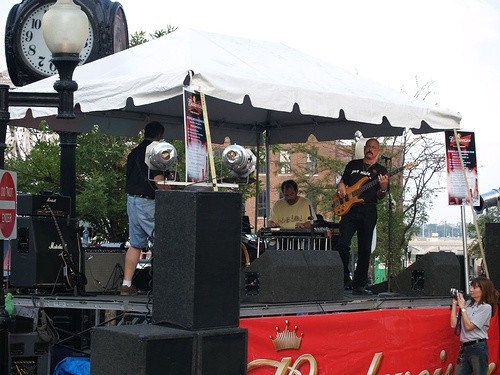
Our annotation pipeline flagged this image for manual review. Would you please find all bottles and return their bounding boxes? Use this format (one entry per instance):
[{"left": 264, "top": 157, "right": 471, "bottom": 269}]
[{"left": 82, "top": 227, "right": 89, "bottom": 246}]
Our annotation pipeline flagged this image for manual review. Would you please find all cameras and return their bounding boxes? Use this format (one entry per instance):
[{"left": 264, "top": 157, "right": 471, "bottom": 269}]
[{"left": 449, "top": 288, "right": 465, "bottom": 300}]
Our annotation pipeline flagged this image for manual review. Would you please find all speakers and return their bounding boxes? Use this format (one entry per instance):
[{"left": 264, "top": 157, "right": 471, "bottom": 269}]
[
  {"left": 91, "top": 190, "right": 250, "bottom": 375},
  {"left": 391, "top": 252, "right": 462, "bottom": 297},
  {"left": 10, "top": 217, "right": 81, "bottom": 291},
  {"left": 242, "top": 249, "right": 344, "bottom": 302},
  {"left": 79, "top": 247, "right": 127, "bottom": 293}
]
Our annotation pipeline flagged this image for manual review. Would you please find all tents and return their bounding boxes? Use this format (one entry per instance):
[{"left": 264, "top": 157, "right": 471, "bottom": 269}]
[{"left": 0, "top": 28, "right": 471, "bottom": 297}]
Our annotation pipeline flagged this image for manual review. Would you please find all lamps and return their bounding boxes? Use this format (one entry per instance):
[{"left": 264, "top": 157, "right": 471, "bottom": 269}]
[
  {"left": 144, "top": 139, "right": 178, "bottom": 183},
  {"left": 473, "top": 188, "right": 500, "bottom": 214},
  {"left": 218, "top": 145, "right": 257, "bottom": 185}
]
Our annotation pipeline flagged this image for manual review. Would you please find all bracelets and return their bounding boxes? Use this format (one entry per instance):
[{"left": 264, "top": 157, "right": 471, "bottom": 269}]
[
  {"left": 380, "top": 189, "right": 387, "bottom": 192},
  {"left": 461, "top": 308, "right": 466, "bottom": 312}
]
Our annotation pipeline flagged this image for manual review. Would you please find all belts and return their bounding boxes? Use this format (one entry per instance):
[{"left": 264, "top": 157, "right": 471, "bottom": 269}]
[
  {"left": 129, "top": 194, "right": 155, "bottom": 200},
  {"left": 463, "top": 338, "right": 487, "bottom": 346}
]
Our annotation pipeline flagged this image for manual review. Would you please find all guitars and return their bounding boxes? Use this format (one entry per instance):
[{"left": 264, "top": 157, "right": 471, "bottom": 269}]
[
  {"left": 332, "top": 161, "right": 418, "bottom": 217},
  {"left": 42, "top": 201, "right": 88, "bottom": 289}
]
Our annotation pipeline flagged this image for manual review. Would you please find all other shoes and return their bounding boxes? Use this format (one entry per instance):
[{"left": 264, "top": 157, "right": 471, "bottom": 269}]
[
  {"left": 120, "top": 284, "right": 138, "bottom": 296},
  {"left": 351, "top": 286, "right": 373, "bottom": 296}
]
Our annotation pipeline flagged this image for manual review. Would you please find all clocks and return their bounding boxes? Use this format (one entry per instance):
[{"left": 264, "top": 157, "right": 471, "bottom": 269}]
[{"left": 18, "top": 2, "right": 94, "bottom": 77}]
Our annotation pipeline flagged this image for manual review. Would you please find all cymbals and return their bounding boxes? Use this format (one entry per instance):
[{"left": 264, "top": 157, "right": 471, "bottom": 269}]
[
  {"left": 303, "top": 219, "right": 327, "bottom": 227},
  {"left": 217, "top": 177, "right": 257, "bottom": 186}
]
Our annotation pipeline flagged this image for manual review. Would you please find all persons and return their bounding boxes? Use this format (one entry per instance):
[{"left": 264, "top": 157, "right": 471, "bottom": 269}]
[
  {"left": 450, "top": 277, "right": 497, "bottom": 375},
  {"left": 338, "top": 138, "right": 389, "bottom": 294},
  {"left": 268, "top": 180, "right": 317, "bottom": 227},
  {"left": 119, "top": 121, "right": 167, "bottom": 296}
]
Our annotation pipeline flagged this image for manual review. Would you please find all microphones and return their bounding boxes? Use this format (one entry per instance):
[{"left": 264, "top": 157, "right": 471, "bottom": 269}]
[{"left": 381, "top": 155, "right": 391, "bottom": 160}]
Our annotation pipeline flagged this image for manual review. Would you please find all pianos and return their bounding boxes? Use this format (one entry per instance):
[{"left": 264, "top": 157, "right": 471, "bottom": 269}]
[{"left": 256, "top": 225, "right": 333, "bottom": 260}]
